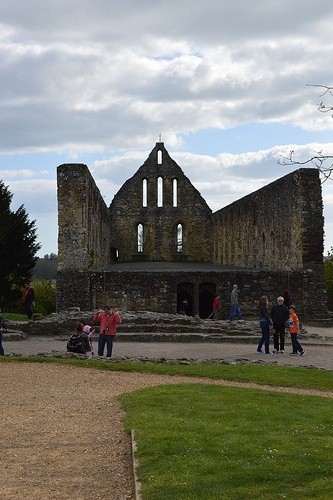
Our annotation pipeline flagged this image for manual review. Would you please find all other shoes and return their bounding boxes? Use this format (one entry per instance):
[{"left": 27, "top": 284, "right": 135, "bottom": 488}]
[
  {"left": 281, "top": 350, "right": 284, "bottom": 354},
  {"left": 300, "top": 351, "right": 306, "bottom": 356},
  {"left": 288, "top": 352, "right": 297, "bottom": 356},
  {"left": 272, "top": 349, "right": 279, "bottom": 353},
  {"left": 264, "top": 352, "right": 274, "bottom": 355},
  {"left": 257, "top": 350, "right": 264, "bottom": 353}
]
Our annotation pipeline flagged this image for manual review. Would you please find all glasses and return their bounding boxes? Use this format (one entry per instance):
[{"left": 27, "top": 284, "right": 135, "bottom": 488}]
[
  {"left": 278, "top": 300, "right": 283, "bottom": 302},
  {"left": 24, "top": 285, "right": 28, "bottom": 286}
]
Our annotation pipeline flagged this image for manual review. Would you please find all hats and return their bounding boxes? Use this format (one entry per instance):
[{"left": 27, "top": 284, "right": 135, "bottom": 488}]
[{"left": 289, "top": 305, "right": 295, "bottom": 309}]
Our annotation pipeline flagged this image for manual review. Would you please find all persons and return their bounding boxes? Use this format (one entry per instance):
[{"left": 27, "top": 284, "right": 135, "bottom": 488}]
[
  {"left": 256, "top": 295, "right": 273, "bottom": 355},
  {"left": 0, "top": 312, "right": 8, "bottom": 358},
  {"left": 213, "top": 295, "right": 221, "bottom": 320},
  {"left": 288, "top": 304, "right": 306, "bottom": 356},
  {"left": 226, "top": 284, "right": 245, "bottom": 322},
  {"left": 65, "top": 322, "right": 95, "bottom": 359},
  {"left": 270, "top": 296, "right": 289, "bottom": 354},
  {"left": 93, "top": 304, "right": 121, "bottom": 361},
  {"left": 179, "top": 298, "right": 189, "bottom": 317},
  {"left": 21, "top": 282, "right": 36, "bottom": 320}
]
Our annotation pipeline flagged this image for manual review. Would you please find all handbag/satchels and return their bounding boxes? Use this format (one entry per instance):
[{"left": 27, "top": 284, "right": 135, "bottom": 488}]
[{"left": 19, "top": 297, "right": 26, "bottom": 311}]
